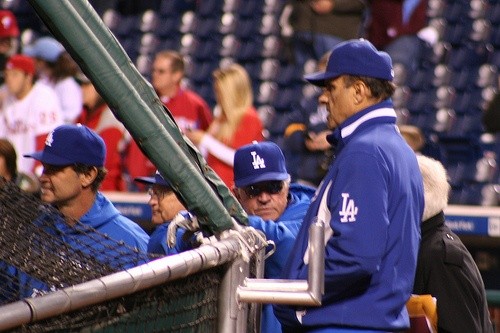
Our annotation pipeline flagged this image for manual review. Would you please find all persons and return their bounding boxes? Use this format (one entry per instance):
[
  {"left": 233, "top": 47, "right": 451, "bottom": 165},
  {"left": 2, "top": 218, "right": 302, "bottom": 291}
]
[
  {"left": 17, "top": 122, "right": 152, "bottom": 299},
  {"left": 182, "top": 62, "right": 263, "bottom": 200},
  {"left": 166, "top": 143, "right": 318, "bottom": 333},
  {"left": 119, "top": 49, "right": 214, "bottom": 191},
  {"left": 0, "top": 1, "right": 500, "bottom": 333},
  {"left": 273, "top": 38, "right": 425, "bottom": 333}
]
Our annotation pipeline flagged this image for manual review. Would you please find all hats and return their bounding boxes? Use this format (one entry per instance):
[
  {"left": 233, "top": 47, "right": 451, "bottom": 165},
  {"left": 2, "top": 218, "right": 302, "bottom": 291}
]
[
  {"left": 304, "top": 38, "right": 395, "bottom": 88},
  {"left": 5, "top": 54, "right": 35, "bottom": 76},
  {"left": 133, "top": 168, "right": 170, "bottom": 186},
  {"left": 22, "top": 122, "right": 105, "bottom": 167},
  {"left": 20, "top": 36, "right": 67, "bottom": 62},
  {"left": 0, "top": 9, "right": 19, "bottom": 40},
  {"left": 234, "top": 139, "right": 288, "bottom": 187}
]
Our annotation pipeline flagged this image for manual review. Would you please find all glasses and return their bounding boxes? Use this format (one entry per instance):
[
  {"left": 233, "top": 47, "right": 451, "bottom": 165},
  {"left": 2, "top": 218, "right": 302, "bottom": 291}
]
[
  {"left": 246, "top": 180, "right": 284, "bottom": 198},
  {"left": 151, "top": 66, "right": 167, "bottom": 75},
  {"left": 147, "top": 183, "right": 171, "bottom": 203}
]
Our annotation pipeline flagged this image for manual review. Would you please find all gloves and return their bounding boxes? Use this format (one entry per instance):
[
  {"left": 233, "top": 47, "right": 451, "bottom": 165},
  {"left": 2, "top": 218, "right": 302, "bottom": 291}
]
[{"left": 165, "top": 213, "right": 198, "bottom": 249}]
